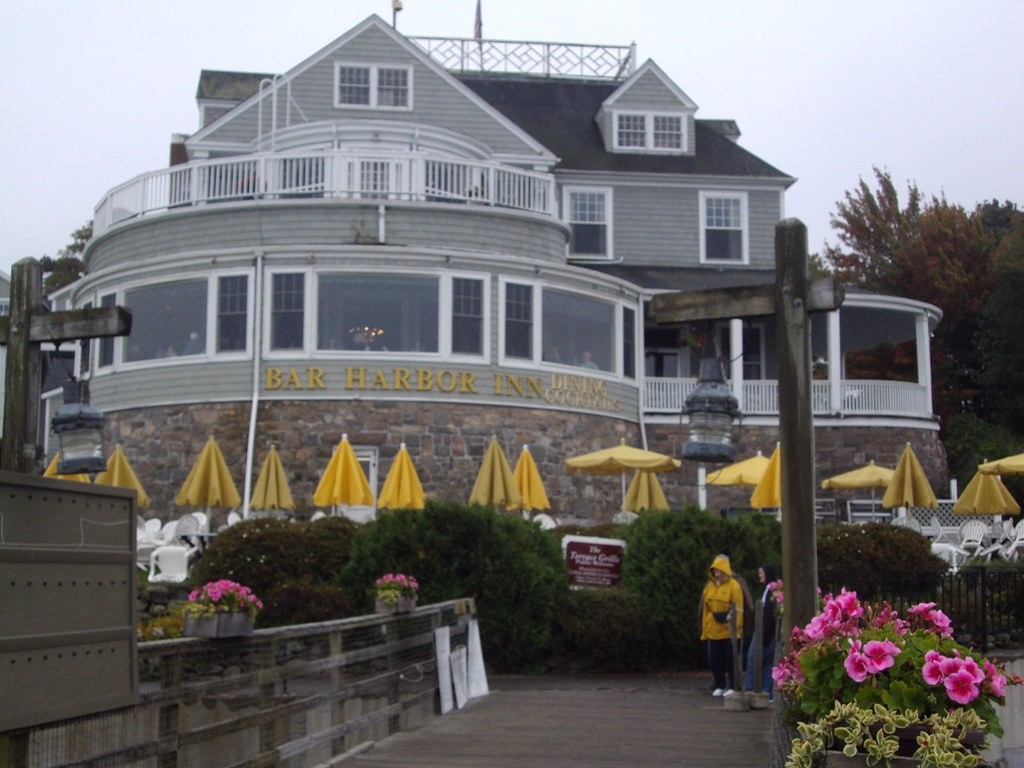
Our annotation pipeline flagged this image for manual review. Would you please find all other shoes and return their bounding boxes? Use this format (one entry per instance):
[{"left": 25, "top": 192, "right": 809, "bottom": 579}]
[{"left": 712, "top": 688, "right": 737, "bottom": 697}]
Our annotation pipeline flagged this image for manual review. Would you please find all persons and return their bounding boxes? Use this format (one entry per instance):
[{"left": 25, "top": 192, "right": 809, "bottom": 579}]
[
  {"left": 697, "top": 554, "right": 754, "bottom": 697},
  {"left": 579, "top": 351, "right": 597, "bottom": 369},
  {"left": 745, "top": 564, "right": 781, "bottom": 704}
]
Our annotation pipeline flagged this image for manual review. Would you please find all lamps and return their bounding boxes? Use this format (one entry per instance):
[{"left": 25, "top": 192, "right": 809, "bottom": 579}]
[
  {"left": 46, "top": 374, "right": 109, "bottom": 478},
  {"left": 677, "top": 347, "right": 743, "bottom": 464}
]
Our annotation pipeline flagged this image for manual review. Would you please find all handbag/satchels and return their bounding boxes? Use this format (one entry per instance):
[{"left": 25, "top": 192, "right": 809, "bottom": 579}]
[{"left": 711, "top": 611, "right": 730, "bottom": 625}]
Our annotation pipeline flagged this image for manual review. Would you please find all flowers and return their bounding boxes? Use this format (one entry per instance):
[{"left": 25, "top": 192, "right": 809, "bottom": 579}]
[
  {"left": 179, "top": 577, "right": 269, "bottom": 625},
  {"left": 372, "top": 570, "right": 419, "bottom": 604},
  {"left": 771, "top": 584, "right": 1024, "bottom": 768}
]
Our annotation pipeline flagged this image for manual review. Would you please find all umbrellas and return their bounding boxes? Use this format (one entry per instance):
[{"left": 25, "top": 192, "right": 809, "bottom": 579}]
[
  {"left": 43, "top": 452, "right": 90, "bottom": 482},
  {"left": 822, "top": 459, "right": 895, "bottom": 521},
  {"left": 707, "top": 450, "right": 770, "bottom": 513},
  {"left": 249, "top": 444, "right": 295, "bottom": 512},
  {"left": 622, "top": 468, "right": 669, "bottom": 513},
  {"left": 505, "top": 444, "right": 550, "bottom": 520},
  {"left": 93, "top": 443, "right": 150, "bottom": 508},
  {"left": 377, "top": 442, "right": 425, "bottom": 509},
  {"left": 954, "top": 458, "right": 1020, "bottom": 526},
  {"left": 977, "top": 453, "right": 1024, "bottom": 475},
  {"left": 176, "top": 435, "right": 241, "bottom": 512},
  {"left": 566, "top": 438, "right": 681, "bottom": 512},
  {"left": 751, "top": 441, "right": 781, "bottom": 518},
  {"left": 313, "top": 433, "right": 374, "bottom": 516},
  {"left": 469, "top": 435, "right": 521, "bottom": 505},
  {"left": 882, "top": 442, "right": 936, "bottom": 515}
]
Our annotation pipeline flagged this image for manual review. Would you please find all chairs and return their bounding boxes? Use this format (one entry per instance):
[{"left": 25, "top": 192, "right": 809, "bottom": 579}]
[
  {"left": 888, "top": 514, "right": 1024, "bottom": 575},
  {"left": 135, "top": 503, "right": 239, "bottom": 584}
]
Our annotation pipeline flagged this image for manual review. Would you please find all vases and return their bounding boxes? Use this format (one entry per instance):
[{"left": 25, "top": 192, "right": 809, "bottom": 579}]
[
  {"left": 184, "top": 608, "right": 256, "bottom": 640},
  {"left": 374, "top": 595, "right": 418, "bottom": 613},
  {"left": 812, "top": 748, "right": 994, "bottom": 768}
]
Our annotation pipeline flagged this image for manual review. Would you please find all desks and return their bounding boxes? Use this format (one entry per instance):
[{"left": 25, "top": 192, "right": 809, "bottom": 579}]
[
  {"left": 178, "top": 529, "right": 221, "bottom": 563},
  {"left": 983, "top": 531, "right": 1013, "bottom": 559}
]
